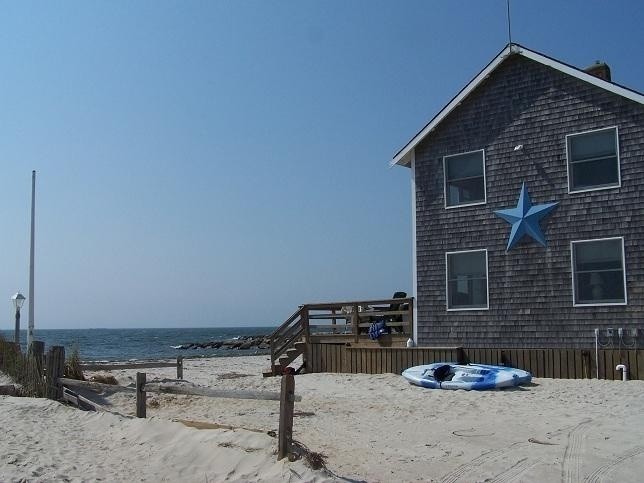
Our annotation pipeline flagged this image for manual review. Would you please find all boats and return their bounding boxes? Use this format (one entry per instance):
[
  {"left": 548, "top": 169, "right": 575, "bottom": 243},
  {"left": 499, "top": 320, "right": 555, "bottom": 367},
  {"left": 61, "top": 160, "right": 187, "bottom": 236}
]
[{"left": 400, "top": 358, "right": 536, "bottom": 392}]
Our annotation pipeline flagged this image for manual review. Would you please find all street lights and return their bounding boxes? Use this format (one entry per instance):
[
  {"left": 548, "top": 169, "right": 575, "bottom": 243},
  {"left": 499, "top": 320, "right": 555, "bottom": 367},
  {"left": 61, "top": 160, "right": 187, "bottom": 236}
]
[{"left": 11, "top": 292, "right": 26, "bottom": 345}]
